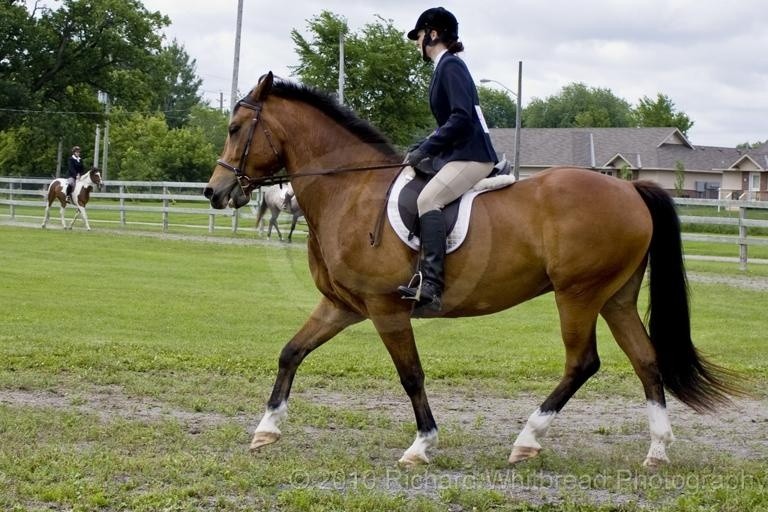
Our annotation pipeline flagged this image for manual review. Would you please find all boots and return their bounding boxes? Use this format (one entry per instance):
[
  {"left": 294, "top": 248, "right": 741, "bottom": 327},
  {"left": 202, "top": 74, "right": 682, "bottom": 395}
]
[
  {"left": 66, "top": 185, "right": 73, "bottom": 203},
  {"left": 397, "top": 210, "right": 447, "bottom": 303}
]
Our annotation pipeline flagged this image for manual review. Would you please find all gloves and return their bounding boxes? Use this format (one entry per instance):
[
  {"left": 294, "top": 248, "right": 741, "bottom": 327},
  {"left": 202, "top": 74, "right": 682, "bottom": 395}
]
[
  {"left": 408, "top": 142, "right": 421, "bottom": 153},
  {"left": 408, "top": 148, "right": 424, "bottom": 166}
]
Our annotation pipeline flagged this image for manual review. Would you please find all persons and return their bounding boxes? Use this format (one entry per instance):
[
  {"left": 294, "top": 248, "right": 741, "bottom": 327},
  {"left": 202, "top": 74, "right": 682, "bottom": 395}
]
[
  {"left": 280, "top": 186, "right": 294, "bottom": 209},
  {"left": 65, "top": 146, "right": 86, "bottom": 200},
  {"left": 395, "top": 6, "right": 500, "bottom": 309}
]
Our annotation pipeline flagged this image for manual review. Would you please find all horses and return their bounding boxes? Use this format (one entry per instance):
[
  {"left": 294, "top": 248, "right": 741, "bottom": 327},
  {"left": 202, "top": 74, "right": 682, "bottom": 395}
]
[
  {"left": 253, "top": 183, "right": 306, "bottom": 243},
  {"left": 40, "top": 166, "right": 105, "bottom": 231},
  {"left": 202, "top": 69, "right": 750, "bottom": 471}
]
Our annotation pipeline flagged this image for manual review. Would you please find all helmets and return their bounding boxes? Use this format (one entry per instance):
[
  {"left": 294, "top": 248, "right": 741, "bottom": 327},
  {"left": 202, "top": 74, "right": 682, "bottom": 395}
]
[
  {"left": 72, "top": 146, "right": 80, "bottom": 154},
  {"left": 407, "top": 7, "right": 458, "bottom": 40}
]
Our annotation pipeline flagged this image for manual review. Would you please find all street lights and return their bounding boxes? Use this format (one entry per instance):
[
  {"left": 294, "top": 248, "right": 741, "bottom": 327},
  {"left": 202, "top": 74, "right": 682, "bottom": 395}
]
[{"left": 480, "top": 79, "right": 522, "bottom": 179}]
